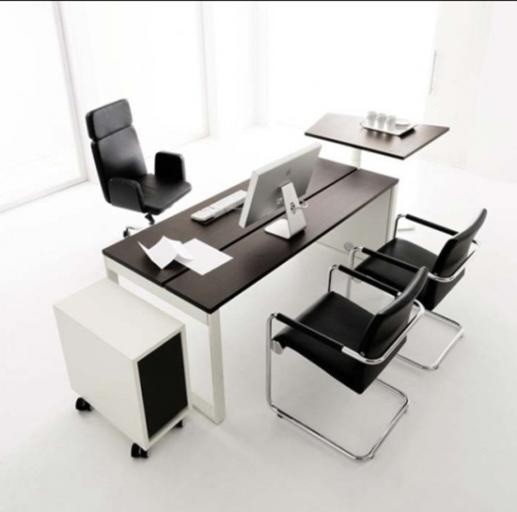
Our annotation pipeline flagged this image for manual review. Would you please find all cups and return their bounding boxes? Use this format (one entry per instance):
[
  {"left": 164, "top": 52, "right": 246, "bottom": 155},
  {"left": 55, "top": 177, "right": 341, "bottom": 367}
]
[{"left": 365, "top": 111, "right": 396, "bottom": 130}]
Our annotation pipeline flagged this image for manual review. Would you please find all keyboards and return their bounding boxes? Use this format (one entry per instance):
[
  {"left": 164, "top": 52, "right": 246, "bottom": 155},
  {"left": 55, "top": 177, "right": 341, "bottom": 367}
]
[{"left": 189, "top": 188, "right": 248, "bottom": 226}]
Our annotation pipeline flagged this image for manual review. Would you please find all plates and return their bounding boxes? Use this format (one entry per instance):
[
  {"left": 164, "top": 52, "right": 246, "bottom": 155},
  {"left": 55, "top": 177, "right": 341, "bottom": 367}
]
[{"left": 360, "top": 118, "right": 417, "bottom": 136}]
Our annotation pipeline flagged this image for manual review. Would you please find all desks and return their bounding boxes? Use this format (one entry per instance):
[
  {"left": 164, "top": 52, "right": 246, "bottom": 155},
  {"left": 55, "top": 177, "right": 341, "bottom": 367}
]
[
  {"left": 304, "top": 113, "right": 449, "bottom": 169},
  {"left": 100, "top": 158, "right": 398, "bottom": 426}
]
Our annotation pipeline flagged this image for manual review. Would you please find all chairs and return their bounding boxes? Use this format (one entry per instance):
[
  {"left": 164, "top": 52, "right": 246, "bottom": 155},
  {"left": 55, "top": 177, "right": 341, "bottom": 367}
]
[
  {"left": 269, "top": 261, "right": 432, "bottom": 464},
  {"left": 345, "top": 207, "right": 489, "bottom": 374},
  {"left": 84, "top": 99, "right": 192, "bottom": 237}
]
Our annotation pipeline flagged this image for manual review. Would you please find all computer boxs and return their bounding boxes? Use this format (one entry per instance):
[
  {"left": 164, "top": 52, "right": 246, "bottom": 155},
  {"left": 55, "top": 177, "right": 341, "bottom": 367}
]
[{"left": 50, "top": 277, "right": 195, "bottom": 452}]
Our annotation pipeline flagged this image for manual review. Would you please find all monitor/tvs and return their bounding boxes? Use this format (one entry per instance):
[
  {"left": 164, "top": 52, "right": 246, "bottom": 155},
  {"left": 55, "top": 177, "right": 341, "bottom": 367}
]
[{"left": 239, "top": 141, "right": 322, "bottom": 240}]
